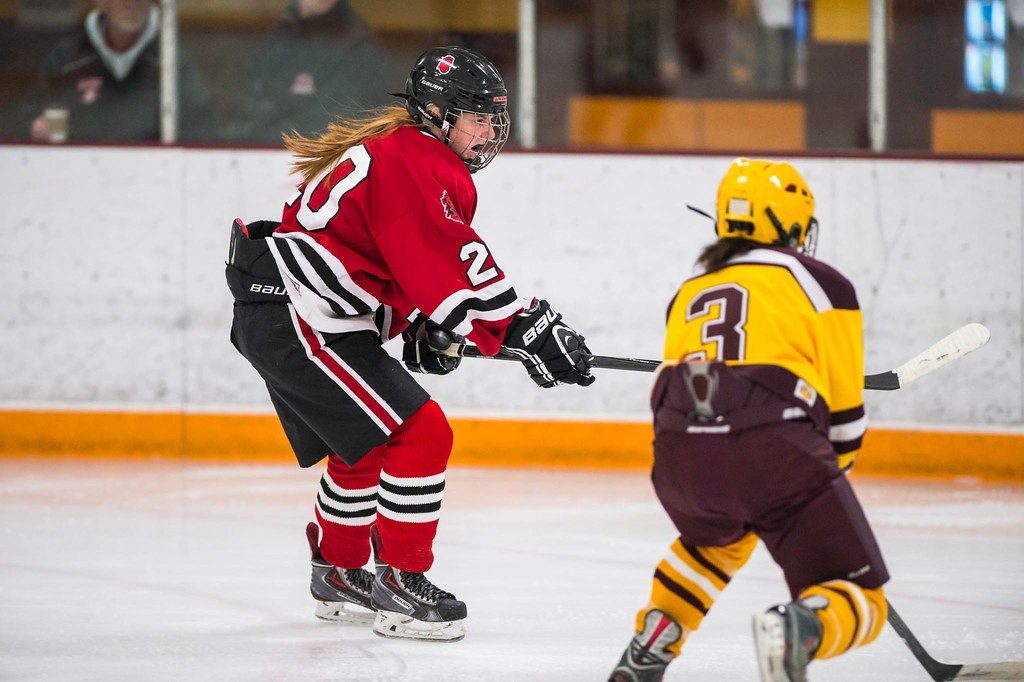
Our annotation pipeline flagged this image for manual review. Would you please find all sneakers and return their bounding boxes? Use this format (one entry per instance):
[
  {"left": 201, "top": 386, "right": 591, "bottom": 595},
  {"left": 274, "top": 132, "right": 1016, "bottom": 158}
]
[
  {"left": 305, "top": 522, "right": 378, "bottom": 624},
  {"left": 369, "top": 523, "right": 467, "bottom": 641},
  {"left": 606, "top": 609, "right": 682, "bottom": 682},
  {"left": 753, "top": 594, "right": 830, "bottom": 682}
]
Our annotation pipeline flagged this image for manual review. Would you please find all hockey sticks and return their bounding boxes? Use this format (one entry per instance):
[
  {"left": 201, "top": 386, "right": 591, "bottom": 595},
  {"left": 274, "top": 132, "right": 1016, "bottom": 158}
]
[
  {"left": 885, "top": 600, "right": 1024, "bottom": 682},
  {"left": 441, "top": 321, "right": 990, "bottom": 391}
]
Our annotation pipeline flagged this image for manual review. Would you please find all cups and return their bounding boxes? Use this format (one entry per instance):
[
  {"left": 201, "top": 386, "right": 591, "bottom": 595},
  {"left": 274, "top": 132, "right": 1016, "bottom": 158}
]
[{"left": 44, "top": 109, "right": 68, "bottom": 143}]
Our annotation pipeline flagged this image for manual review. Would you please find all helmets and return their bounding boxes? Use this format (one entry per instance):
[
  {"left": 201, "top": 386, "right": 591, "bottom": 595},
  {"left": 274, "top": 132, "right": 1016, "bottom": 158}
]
[
  {"left": 716, "top": 157, "right": 819, "bottom": 260},
  {"left": 388, "top": 46, "right": 510, "bottom": 173}
]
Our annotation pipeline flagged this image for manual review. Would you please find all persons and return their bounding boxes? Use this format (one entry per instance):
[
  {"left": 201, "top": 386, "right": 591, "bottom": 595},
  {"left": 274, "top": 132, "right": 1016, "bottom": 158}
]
[
  {"left": 204, "top": 0, "right": 387, "bottom": 143},
  {"left": 798, "top": 0, "right": 962, "bottom": 153},
  {"left": 606, "top": 156, "right": 891, "bottom": 682},
  {"left": 228, "top": 47, "right": 596, "bottom": 644},
  {"left": 17, "top": 0, "right": 209, "bottom": 143}
]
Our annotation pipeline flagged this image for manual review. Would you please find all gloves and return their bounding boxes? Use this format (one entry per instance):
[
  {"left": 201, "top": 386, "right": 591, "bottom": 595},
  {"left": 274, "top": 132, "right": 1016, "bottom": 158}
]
[
  {"left": 498, "top": 297, "right": 595, "bottom": 388},
  {"left": 402, "top": 312, "right": 462, "bottom": 375}
]
